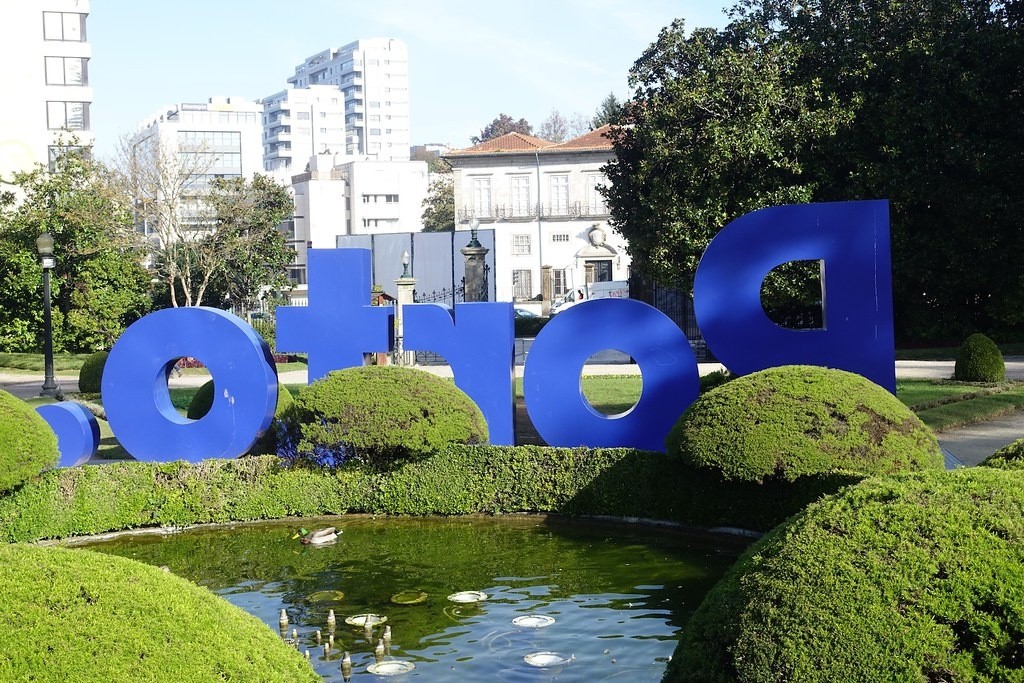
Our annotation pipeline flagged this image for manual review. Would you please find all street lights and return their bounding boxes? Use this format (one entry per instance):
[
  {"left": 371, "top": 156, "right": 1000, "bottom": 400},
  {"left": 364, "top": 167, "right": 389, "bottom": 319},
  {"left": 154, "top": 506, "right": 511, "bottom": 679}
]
[{"left": 37, "top": 233, "right": 58, "bottom": 394}]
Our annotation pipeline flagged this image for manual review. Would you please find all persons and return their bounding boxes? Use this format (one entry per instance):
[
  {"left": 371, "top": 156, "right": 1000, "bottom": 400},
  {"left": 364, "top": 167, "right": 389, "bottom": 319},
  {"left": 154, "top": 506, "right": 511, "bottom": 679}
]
[
  {"left": 577, "top": 290, "right": 584, "bottom": 299},
  {"left": 169, "top": 365, "right": 182, "bottom": 379}
]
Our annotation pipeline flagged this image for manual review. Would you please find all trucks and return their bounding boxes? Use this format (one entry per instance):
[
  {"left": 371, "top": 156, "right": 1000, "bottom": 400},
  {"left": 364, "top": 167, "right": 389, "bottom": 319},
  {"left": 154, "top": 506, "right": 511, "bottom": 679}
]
[{"left": 551, "top": 280, "right": 628, "bottom": 319}]
